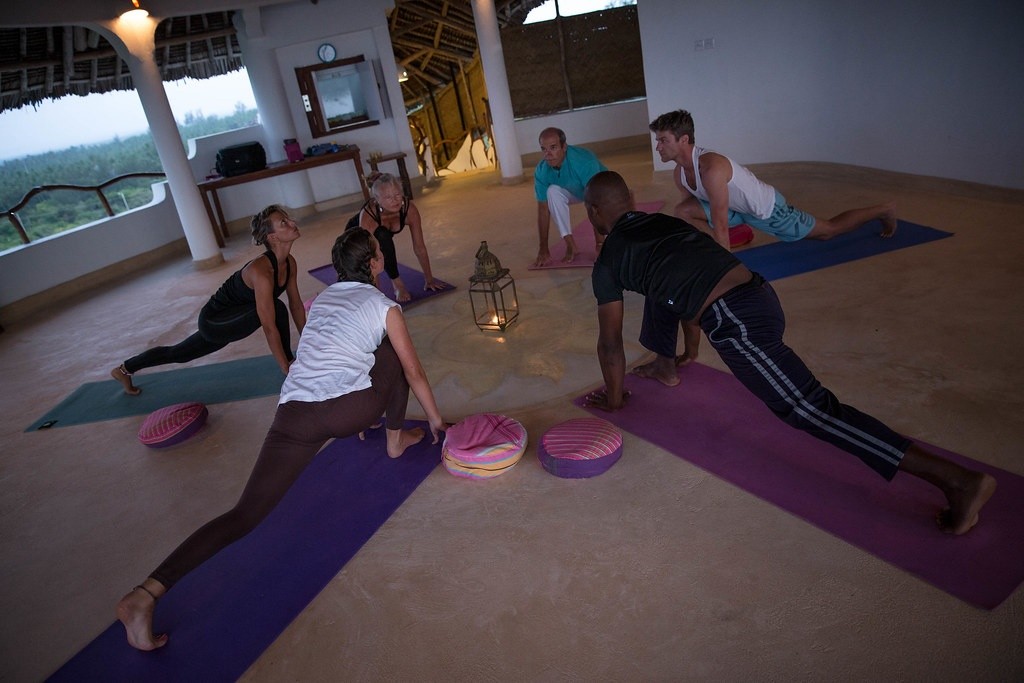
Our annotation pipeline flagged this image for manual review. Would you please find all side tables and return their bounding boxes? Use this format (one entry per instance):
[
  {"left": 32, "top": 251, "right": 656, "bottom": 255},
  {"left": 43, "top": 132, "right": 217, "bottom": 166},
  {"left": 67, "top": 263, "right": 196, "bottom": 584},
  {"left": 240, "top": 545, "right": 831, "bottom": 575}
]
[{"left": 366, "top": 152, "right": 414, "bottom": 201}]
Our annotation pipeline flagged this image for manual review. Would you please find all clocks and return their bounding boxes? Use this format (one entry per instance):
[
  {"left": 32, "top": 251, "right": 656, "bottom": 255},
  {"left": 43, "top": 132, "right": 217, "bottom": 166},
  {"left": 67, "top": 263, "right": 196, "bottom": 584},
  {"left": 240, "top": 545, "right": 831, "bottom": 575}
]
[{"left": 318, "top": 44, "right": 336, "bottom": 64}]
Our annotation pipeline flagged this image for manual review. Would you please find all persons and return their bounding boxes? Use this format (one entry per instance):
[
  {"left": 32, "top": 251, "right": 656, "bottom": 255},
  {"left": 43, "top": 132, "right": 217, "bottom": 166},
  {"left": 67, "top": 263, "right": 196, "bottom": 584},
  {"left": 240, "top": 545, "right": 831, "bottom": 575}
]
[
  {"left": 110, "top": 205, "right": 306, "bottom": 394},
  {"left": 114, "top": 228, "right": 451, "bottom": 652},
  {"left": 581, "top": 171, "right": 998, "bottom": 538},
  {"left": 532, "top": 127, "right": 609, "bottom": 269},
  {"left": 647, "top": 110, "right": 898, "bottom": 254},
  {"left": 330, "top": 171, "right": 447, "bottom": 302}
]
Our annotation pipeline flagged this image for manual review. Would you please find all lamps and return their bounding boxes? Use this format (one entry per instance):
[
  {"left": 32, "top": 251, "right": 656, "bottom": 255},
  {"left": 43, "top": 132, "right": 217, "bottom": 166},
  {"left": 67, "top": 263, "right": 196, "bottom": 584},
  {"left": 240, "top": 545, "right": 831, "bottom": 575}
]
[
  {"left": 395, "top": 61, "right": 408, "bottom": 82},
  {"left": 468, "top": 241, "right": 520, "bottom": 333}
]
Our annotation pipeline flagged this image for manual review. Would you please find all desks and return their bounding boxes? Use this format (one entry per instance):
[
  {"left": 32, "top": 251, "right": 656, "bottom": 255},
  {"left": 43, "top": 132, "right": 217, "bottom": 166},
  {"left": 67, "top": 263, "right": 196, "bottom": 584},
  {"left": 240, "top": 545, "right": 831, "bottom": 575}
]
[{"left": 197, "top": 145, "right": 372, "bottom": 248}]
